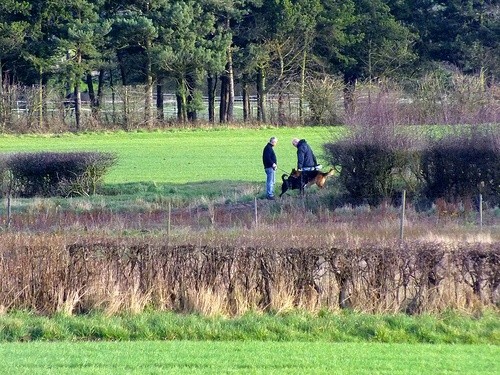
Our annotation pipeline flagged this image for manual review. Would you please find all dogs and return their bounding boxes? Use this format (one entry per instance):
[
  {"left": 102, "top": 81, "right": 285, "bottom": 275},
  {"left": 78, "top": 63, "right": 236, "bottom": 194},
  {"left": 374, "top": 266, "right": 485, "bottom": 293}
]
[
  {"left": 290, "top": 168, "right": 334, "bottom": 189},
  {"left": 279, "top": 173, "right": 310, "bottom": 199}
]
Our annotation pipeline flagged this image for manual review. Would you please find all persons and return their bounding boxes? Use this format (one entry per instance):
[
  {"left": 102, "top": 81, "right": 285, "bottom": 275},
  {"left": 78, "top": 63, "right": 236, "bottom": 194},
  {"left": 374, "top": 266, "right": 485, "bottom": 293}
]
[
  {"left": 291, "top": 138, "right": 319, "bottom": 184},
  {"left": 262, "top": 136, "right": 278, "bottom": 199}
]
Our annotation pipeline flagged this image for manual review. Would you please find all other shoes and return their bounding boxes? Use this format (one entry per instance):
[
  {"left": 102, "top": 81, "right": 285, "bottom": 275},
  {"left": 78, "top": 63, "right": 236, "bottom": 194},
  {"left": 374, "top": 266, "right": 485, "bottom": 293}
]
[{"left": 267, "top": 195, "right": 275, "bottom": 200}]
